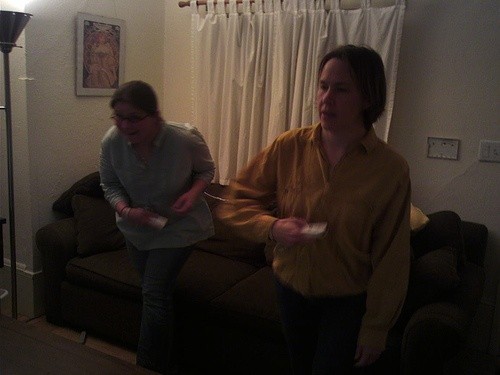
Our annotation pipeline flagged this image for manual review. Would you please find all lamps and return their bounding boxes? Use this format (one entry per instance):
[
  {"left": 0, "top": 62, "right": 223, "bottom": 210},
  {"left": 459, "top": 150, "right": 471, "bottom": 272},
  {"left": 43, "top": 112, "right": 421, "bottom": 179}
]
[{"left": 0, "top": 9, "right": 33, "bottom": 318}]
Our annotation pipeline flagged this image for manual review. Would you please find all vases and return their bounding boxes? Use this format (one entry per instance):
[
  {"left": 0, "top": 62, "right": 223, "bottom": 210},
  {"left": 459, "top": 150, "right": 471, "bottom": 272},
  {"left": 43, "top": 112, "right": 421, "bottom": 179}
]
[{"left": 0, "top": 287, "right": 7, "bottom": 310}]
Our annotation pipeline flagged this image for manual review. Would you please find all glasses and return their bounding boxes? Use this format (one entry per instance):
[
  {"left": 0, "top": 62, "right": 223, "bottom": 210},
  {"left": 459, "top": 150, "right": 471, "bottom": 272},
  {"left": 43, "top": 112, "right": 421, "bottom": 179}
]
[{"left": 110, "top": 111, "right": 152, "bottom": 122}]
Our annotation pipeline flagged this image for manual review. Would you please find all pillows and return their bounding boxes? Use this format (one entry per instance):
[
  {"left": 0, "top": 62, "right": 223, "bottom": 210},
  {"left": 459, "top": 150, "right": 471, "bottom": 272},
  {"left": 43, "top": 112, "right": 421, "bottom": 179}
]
[
  {"left": 413, "top": 249, "right": 468, "bottom": 305},
  {"left": 408, "top": 210, "right": 464, "bottom": 251},
  {"left": 52, "top": 172, "right": 102, "bottom": 217},
  {"left": 71, "top": 194, "right": 126, "bottom": 257}
]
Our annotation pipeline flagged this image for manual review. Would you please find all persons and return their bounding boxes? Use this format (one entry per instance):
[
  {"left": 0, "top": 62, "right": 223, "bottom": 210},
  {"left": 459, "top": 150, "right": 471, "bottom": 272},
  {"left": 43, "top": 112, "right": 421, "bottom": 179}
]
[
  {"left": 219, "top": 43, "right": 412, "bottom": 373},
  {"left": 99, "top": 81, "right": 217, "bottom": 374}
]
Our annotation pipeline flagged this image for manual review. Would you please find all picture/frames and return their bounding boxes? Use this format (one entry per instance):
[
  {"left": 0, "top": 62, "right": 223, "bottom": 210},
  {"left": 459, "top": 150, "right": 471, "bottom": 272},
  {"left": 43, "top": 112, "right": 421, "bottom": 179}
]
[{"left": 75, "top": 12, "right": 127, "bottom": 97}]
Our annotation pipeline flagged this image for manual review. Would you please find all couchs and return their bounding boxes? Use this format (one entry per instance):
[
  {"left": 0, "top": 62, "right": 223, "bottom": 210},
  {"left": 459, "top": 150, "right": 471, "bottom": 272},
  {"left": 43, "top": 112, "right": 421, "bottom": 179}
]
[{"left": 35, "top": 169, "right": 488, "bottom": 375}]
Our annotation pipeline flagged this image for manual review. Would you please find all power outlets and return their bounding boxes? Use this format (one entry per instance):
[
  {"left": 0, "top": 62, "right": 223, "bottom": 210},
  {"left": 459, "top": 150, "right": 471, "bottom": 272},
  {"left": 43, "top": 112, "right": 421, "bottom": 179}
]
[{"left": 478, "top": 139, "right": 500, "bottom": 164}]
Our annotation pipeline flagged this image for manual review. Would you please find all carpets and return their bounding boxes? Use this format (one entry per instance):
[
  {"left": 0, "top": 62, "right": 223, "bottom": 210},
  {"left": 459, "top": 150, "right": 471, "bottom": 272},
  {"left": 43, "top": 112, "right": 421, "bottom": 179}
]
[{"left": 1, "top": 314, "right": 158, "bottom": 375}]
